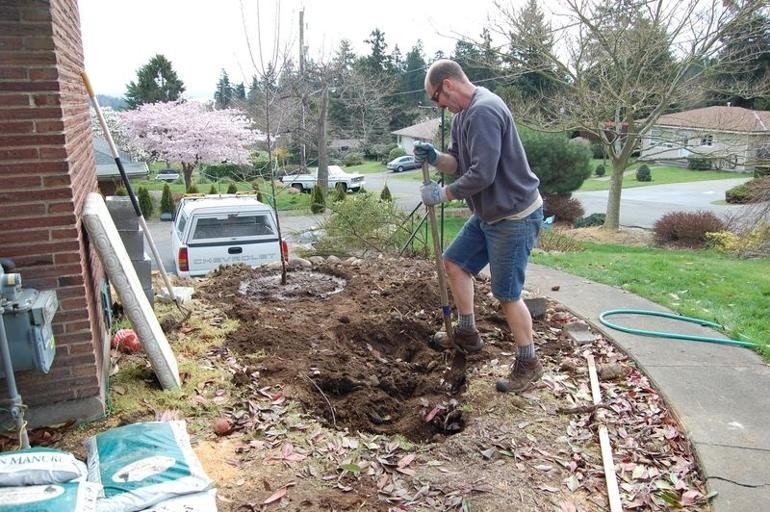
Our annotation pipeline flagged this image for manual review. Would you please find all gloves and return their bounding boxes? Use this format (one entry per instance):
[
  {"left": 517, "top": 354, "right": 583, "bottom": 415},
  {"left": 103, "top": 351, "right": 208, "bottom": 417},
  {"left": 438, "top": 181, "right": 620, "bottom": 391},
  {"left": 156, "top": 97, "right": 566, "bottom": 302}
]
[
  {"left": 412, "top": 140, "right": 439, "bottom": 169},
  {"left": 419, "top": 178, "right": 451, "bottom": 208}
]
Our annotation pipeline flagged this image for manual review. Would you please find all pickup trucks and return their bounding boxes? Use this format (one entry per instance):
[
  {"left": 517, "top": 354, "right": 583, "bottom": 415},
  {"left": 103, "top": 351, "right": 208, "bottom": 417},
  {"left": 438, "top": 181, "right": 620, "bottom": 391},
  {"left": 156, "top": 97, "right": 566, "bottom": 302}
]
[{"left": 282, "top": 166, "right": 368, "bottom": 193}]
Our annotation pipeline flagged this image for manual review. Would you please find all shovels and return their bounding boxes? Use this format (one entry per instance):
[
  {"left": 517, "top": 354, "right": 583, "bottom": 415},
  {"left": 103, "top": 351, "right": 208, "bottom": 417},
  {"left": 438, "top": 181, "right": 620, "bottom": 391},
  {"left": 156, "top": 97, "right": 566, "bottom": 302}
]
[{"left": 422, "top": 160, "right": 465, "bottom": 385}]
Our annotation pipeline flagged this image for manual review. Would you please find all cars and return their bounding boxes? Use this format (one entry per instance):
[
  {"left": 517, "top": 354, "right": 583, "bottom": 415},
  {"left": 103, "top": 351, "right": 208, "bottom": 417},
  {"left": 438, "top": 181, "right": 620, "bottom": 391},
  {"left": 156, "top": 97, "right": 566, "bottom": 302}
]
[
  {"left": 155, "top": 167, "right": 181, "bottom": 182},
  {"left": 386, "top": 155, "right": 422, "bottom": 172},
  {"left": 169, "top": 193, "right": 289, "bottom": 278}
]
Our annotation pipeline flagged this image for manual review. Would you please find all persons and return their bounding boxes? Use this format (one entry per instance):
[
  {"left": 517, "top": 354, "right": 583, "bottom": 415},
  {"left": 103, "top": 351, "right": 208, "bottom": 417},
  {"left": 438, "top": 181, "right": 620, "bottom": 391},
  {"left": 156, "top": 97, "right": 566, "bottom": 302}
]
[{"left": 414, "top": 59, "right": 544, "bottom": 393}]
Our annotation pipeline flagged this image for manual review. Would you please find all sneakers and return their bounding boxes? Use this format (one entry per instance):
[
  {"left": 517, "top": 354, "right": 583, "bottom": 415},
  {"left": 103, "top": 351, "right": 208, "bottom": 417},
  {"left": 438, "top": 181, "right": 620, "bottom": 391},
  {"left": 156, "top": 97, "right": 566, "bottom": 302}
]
[
  {"left": 433, "top": 329, "right": 485, "bottom": 352},
  {"left": 496, "top": 354, "right": 546, "bottom": 394}
]
